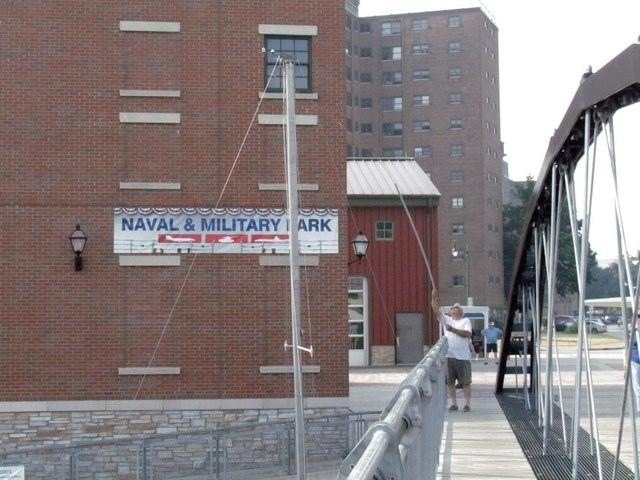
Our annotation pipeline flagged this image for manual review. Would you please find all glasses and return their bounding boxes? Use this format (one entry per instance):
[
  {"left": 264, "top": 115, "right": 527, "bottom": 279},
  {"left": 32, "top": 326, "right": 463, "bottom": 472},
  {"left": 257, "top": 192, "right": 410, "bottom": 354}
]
[{"left": 450, "top": 306, "right": 459, "bottom": 309}]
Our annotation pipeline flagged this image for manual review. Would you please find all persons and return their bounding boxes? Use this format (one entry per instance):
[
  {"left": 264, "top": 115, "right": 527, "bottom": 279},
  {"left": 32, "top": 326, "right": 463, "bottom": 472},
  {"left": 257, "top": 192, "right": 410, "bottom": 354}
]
[
  {"left": 431, "top": 289, "right": 477, "bottom": 412},
  {"left": 628, "top": 314, "right": 640, "bottom": 414},
  {"left": 480, "top": 321, "right": 504, "bottom": 364}
]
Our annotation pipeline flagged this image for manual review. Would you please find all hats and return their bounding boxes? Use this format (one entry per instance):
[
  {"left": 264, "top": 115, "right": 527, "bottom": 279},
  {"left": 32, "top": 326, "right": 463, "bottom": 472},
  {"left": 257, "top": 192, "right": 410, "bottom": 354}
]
[{"left": 488, "top": 322, "right": 494, "bottom": 326}]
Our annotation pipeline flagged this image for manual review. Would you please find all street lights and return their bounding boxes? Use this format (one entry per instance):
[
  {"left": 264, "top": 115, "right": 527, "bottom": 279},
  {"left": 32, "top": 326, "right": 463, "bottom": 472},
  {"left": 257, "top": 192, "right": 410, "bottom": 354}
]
[{"left": 451, "top": 239, "right": 471, "bottom": 305}]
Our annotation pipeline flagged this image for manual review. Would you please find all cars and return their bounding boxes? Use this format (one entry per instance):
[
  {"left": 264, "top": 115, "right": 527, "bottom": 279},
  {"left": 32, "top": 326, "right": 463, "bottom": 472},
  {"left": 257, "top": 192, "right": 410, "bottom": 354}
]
[{"left": 543, "top": 314, "right": 617, "bottom": 333}]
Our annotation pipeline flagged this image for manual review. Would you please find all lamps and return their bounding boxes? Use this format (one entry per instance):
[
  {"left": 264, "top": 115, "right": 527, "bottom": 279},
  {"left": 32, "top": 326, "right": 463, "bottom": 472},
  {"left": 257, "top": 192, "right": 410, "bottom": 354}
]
[{"left": 68, "top": 225, "right": 87, "bottom": 272}]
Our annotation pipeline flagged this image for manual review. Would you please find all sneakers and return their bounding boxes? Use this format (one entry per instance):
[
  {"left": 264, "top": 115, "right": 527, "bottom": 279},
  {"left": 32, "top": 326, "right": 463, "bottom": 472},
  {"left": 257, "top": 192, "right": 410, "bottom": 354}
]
[
  {"left": 484, "top": 361, "right": 499, "bottom": 365},
  {"left": 449, "top": 405, "right": 470, "bottom": 412}
]
